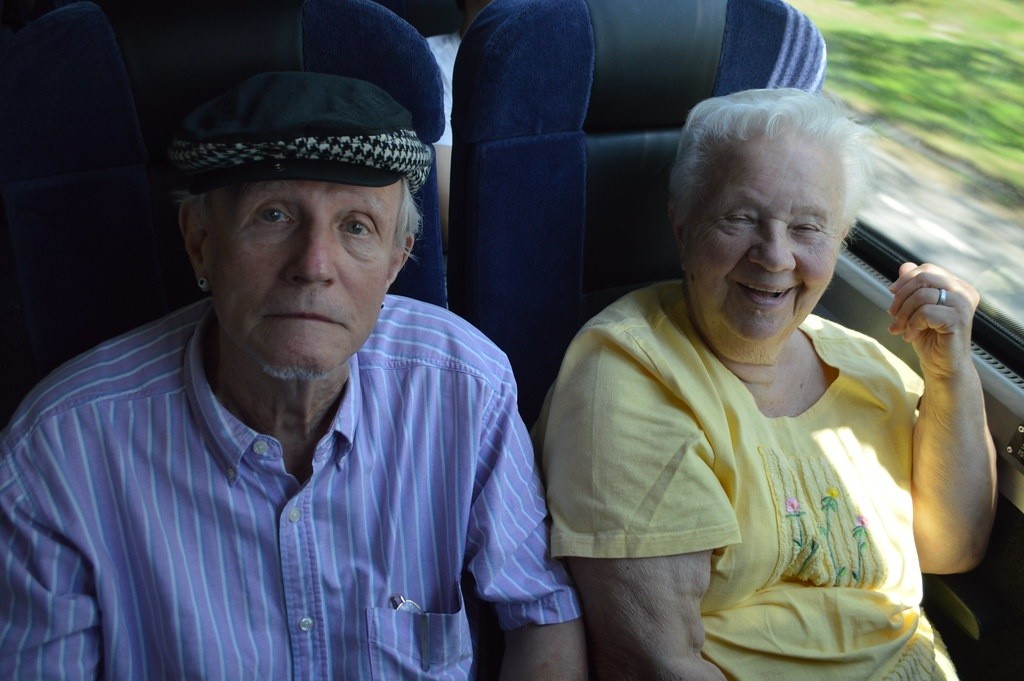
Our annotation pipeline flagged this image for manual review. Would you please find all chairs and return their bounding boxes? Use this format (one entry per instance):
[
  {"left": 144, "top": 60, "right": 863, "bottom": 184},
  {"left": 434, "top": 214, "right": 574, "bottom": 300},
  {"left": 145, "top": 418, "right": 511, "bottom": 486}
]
[
  {"left": 0, "top": 1, "right": 446, "bottom": 431},
  {"left": 447, "top": 1, "right": 827, "bottom": 431}
]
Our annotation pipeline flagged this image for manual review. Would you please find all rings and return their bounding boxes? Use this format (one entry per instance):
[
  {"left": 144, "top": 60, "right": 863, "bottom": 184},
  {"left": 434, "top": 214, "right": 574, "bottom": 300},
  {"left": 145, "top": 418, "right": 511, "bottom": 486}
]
[{"left": 937, "top": 288, "right": 947, "bottom": 305}]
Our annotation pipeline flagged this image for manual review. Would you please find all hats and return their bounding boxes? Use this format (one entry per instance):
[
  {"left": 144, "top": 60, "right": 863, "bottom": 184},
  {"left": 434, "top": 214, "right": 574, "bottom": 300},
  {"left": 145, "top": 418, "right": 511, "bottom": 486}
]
[{"left": 166, "top": 70, "right": 433, "bottom": 195}]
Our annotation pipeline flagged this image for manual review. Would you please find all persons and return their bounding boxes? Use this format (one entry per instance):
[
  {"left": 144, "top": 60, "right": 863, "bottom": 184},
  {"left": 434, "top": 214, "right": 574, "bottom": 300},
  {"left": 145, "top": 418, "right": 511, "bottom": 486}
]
[
  {"left": 0, "top": 69, "right": 591, "bottom": 681},
  {"left": 388, "top": 1, "right": 502, "bottom": 261},
  {"left": 530, "top": 85, "right": 998, "bottom": 681}
]
